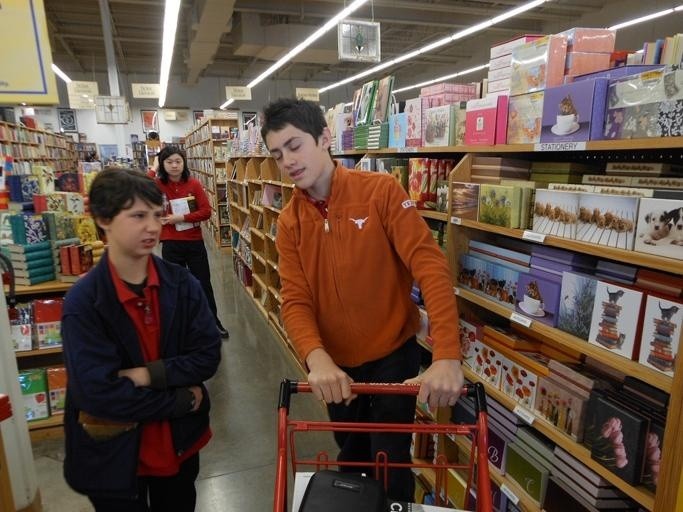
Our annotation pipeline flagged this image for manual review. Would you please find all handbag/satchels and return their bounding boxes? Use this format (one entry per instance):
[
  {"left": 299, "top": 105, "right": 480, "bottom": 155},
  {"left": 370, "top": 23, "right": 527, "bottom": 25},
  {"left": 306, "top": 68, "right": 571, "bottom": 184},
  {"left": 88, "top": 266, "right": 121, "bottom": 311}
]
[{"left": 291, "top": 470, "right": 387, "bottom": 511}]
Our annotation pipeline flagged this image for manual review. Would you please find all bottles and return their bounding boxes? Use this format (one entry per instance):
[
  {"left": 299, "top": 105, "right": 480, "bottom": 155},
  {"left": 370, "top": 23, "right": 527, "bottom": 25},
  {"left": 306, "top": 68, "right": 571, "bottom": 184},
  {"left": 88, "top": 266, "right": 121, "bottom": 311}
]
[{"left": 162, "top": 193, "right": 169, "bottom": 218}]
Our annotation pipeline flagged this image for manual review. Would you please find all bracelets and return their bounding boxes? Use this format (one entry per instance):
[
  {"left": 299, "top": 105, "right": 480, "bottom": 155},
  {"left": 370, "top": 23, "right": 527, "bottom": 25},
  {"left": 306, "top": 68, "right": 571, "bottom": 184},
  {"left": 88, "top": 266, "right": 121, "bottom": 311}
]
[{"left": 188, "top": 390, "right": 195, "bottom": 410}]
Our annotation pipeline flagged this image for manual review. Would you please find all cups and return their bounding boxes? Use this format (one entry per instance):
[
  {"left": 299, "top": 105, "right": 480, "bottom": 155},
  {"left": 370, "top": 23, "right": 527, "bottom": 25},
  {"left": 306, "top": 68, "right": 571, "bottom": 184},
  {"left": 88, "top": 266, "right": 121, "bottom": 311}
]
[
  {"left": 523, "top": 294, "right": 545, "bottom": 313},
  {"left": 557, "top": 114, "right": 579, "bottom": 131}
]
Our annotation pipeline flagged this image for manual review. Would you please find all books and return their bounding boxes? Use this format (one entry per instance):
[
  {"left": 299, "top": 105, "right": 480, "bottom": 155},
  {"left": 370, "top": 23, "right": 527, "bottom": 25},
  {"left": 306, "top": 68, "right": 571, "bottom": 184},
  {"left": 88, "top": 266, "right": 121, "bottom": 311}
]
[
  {"left": 131, "top": 119, "right": 242, "bottom": 287},
  {"left": 0, "top": 118, "right": 96, "bottom": 287}
]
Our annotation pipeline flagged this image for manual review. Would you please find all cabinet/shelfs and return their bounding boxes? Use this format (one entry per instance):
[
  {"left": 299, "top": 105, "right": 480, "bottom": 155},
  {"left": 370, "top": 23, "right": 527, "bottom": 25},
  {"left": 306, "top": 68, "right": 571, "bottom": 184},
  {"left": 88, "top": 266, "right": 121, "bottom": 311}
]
[
  {"left": 0, "top": 119, "right": 151, "bottom": 447},
  {"left": 242, "top": 157, "right": 681, "bottom": 510},
  {"left": 180, "top": 110, "right": 241, "bottom": 249}
]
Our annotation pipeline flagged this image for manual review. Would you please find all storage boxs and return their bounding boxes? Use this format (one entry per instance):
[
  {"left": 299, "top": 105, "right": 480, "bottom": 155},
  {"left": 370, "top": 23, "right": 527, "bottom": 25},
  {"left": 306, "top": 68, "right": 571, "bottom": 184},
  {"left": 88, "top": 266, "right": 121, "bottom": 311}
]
[{"left": 325, "top": 22, "right": 683, "bottom": 146}]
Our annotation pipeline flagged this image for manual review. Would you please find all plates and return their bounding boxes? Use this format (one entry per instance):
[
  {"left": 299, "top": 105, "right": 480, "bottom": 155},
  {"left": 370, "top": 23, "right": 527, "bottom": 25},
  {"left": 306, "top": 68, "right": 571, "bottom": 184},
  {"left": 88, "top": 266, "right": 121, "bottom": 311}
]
[
  {"left": 550, "top": 123, "right": 581, "bottom": 136},
  {"left": 519, "top": 301, "right": 546, "bottom": 317}
]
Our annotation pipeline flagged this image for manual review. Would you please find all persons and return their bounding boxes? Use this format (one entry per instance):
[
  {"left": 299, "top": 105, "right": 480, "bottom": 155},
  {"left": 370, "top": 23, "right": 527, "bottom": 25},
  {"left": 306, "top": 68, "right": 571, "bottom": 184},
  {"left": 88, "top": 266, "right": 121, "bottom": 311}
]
[
  {"left": 57, "top": 168, "right": 219, "bottom": 510},
  {"left": 151, "top": 145, "right": 229, "bottom": 338},
  {"left": 259, "top": 94, "right": 463, "bottom": 500}
]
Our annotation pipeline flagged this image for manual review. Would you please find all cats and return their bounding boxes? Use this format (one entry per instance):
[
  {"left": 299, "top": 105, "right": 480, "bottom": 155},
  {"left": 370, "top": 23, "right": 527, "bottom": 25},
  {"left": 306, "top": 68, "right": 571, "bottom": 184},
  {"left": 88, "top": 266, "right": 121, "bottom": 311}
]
[
  {"left": 606, "top": 285, "right": 626, "bottom": 304},
  {"left": 658, "top": 299, "right": 680, "bottom": 321}
]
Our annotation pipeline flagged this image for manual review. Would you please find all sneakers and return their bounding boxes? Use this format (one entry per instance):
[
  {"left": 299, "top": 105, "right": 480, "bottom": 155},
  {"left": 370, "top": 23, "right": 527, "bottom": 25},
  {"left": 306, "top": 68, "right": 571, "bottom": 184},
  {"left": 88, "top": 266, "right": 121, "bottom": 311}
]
[{"left": 214, "top": 316, "right": 230, "bottom": 340}]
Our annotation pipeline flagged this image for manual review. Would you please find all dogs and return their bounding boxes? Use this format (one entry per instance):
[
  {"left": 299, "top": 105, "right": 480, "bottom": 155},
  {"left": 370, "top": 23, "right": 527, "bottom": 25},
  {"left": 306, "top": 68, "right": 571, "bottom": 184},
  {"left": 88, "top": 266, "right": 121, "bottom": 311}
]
[{"left": 638, "top": 206, "right": 683, "bottom": 247}]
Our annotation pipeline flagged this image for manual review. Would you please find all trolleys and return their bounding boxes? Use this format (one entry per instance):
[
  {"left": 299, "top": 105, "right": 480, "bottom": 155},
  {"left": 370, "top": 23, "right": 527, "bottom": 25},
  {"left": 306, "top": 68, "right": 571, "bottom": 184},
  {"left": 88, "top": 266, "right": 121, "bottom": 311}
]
[{"left": 273, "top": 378, "right": 492, "bottom": 512}]
